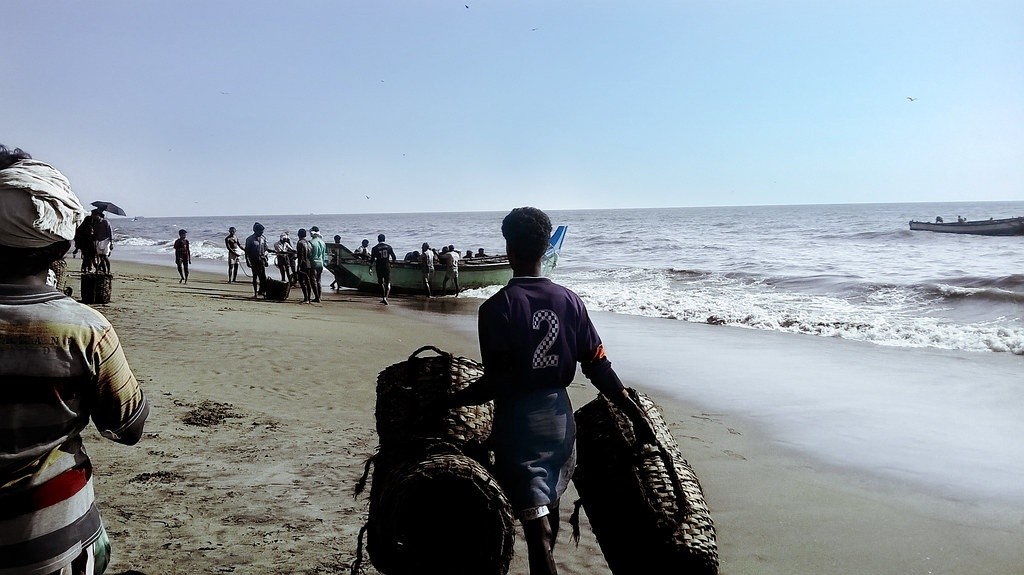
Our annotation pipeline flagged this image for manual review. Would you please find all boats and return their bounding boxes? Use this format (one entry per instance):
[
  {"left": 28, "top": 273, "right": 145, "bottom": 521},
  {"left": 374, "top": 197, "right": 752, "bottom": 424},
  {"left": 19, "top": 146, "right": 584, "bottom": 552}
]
[
  {"left": 909, "top": 216, "right": 1024, "bottom": 237},
  {"left": 323, "top": 226, "right": 568, "bottom": 297}
]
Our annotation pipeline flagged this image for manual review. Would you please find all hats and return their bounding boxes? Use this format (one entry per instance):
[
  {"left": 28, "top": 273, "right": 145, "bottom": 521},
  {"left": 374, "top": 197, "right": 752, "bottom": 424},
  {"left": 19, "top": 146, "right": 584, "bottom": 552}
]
[{"left": 254, "top": 223, "right": 265, "bottom": 231}]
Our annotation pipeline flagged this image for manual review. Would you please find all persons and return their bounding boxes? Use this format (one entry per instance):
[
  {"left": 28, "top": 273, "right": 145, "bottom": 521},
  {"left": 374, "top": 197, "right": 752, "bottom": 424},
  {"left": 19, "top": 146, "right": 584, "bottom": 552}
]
[
  {"left": 245, "top": 222, "right": 269, "bottom": 296},
  {"left": 405, "top": 246, "right": 489, "bottom": 259},
  {"left": 436, "top": 207, "right": 655, "bottom": 575},
  {"left": 435, "top": 245, "right": 460, "bottom": 296},
  {"left": 297, "top": 229, "right": 311, "bottom": 304},
  {"left": 274, "top": 233, "right": 293, "bottom": 286},
  {"left": 225, "top": 227, "right": 245, "bottom": 282},
  {"left": 368, "top": 234, "right": 396, "bottom": 305},
  {"left": 330, "top": 235, "right": 341, "bottom": 287},
  {"left": 418, "top": 242, "right": 438, "bottom": 297},
  {"left": 174, "top": 229, "right": 191, "bottom": 284},
  {"left": 354, "top": 239, "right": 372, "bottom": 255},
  {"left": 308, "top": 226, "right": 326, "bottom": 303},
  {"left": 0, "top": 145, "right": 150, "bottom": 575},
  {"left": 285, "top": 231, "right": 296, "bottom": 272},
  {"left": 74, "top": 207, "right": 113, "bottom": 273}
]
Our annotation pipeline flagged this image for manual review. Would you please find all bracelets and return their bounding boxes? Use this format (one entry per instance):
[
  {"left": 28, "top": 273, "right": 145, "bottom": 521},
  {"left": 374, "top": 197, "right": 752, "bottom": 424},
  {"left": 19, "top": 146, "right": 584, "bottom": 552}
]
[{"left": 111, "top": 243, "right": 113, "bottom": 244}]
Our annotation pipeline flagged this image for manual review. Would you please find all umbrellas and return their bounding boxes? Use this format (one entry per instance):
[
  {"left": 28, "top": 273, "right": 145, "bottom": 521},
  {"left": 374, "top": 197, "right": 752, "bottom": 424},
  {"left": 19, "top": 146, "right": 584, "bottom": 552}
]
[{"left": 91, "top": 201, "right": 126, "bottom": 216}]
